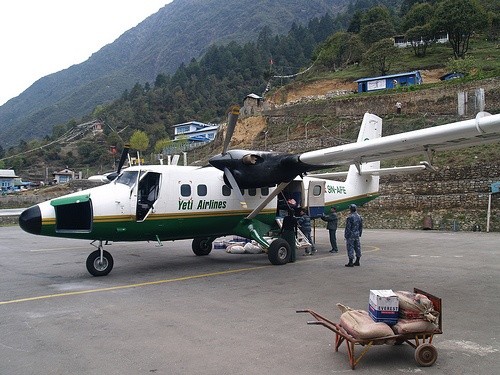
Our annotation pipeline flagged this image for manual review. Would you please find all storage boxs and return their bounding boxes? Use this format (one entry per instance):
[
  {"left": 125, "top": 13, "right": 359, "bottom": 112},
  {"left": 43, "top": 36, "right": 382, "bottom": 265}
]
[{"left": 368, "top": 289, "right": 399, "bottom": 325}]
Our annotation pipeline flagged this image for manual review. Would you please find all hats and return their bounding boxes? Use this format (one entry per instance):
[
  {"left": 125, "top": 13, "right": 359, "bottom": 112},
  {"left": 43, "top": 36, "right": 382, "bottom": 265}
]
[
  {"left": 330, "top": 206, "right": 337, "bottom": 209},
  {"left": 349, "top": 203, "right": 357, "bottom": 211}
]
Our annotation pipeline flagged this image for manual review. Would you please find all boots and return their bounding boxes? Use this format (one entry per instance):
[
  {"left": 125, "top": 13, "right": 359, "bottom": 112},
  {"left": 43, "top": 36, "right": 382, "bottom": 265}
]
[
  {"left": 344, "top": 257, "right": 354, "bottom": 267},
  {"left": 352, "top": 256, "right": 360, "bottom": 266}
]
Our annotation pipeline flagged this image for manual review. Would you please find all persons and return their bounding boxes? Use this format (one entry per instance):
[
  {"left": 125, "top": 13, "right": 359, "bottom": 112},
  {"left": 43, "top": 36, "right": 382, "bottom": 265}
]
[
  {"left": 297, "top": 209, "right": 317, "bottom": 256},
  {"left": 345, "top": 204, "right": 363, "bottom": 267},
  {"left": 393, "top": 80, "right": 397, "bottom": 88},
  {"left": 394, "top": 100, "right": 401, "bottom": 114},
  {"left": 279, "top": 209, "right": 298, "bottom": 263},
  {"left": 321, "top": 206, "right": 338, "bottom": 252}
]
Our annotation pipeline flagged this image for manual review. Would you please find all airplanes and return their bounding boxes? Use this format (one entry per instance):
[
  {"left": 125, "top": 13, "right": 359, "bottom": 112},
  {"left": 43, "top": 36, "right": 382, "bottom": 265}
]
[{"left": 18, "top": 102, "right": 500, "bottom": 278}]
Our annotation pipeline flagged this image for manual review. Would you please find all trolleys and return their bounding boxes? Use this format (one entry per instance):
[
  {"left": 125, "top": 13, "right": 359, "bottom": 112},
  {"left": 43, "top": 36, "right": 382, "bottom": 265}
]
[{"left": 295, "top": 287, "right": 444, "bottom": 371}]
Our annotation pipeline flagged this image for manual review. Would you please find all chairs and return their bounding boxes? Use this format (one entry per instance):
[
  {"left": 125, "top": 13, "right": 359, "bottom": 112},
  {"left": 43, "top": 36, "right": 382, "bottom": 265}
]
[{"left": 137, "top": 184, "right": 158, "bottom": 207}]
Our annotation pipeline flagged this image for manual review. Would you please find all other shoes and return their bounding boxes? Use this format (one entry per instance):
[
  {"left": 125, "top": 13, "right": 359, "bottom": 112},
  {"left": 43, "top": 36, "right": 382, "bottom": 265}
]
[
  {"left": 329, "top": 249, "right": 338, "bottom": 254},
  {"left": 302, "top": 252, "right": 310, "bottom": 256},
  {"left": 310, "top": 249, "right": 317, "bottom": 253}
]
